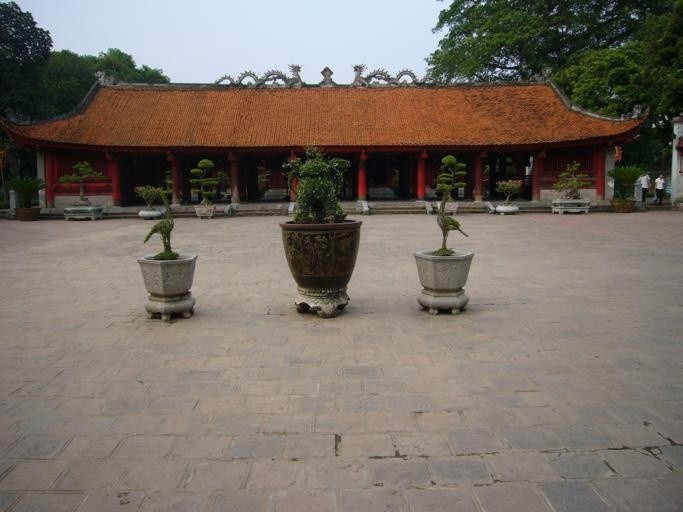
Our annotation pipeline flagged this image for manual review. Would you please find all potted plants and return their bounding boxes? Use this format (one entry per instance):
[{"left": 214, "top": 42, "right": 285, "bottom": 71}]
[
  {"left": 133, "top": 158, "right": 227, "bottom": 324},
  {"left": 550, "top": 161, "right": 592, "bottom": 215},
  {"left": 59, "top": 161, "right": 107, "bottom": 221},
  {"left": 412, "top": 154, "right": 475, "bottom": 317},
  {"left": 607, "top": 165, "right": 646, "bottom": 213},
  {"left": 494, "top": 179, "right": 522, "bottom": 215},
  {"left": 10, "top": 174, "right": 49, "bottom": 220},
  {"left": 277, "top": 144, "right": 363, "bottom": 319}
]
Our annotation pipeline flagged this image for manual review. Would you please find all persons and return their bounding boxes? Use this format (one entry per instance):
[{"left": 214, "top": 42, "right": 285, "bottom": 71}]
[{"left": 637, "top": 173, "right": 666, "bottom": 206}]
